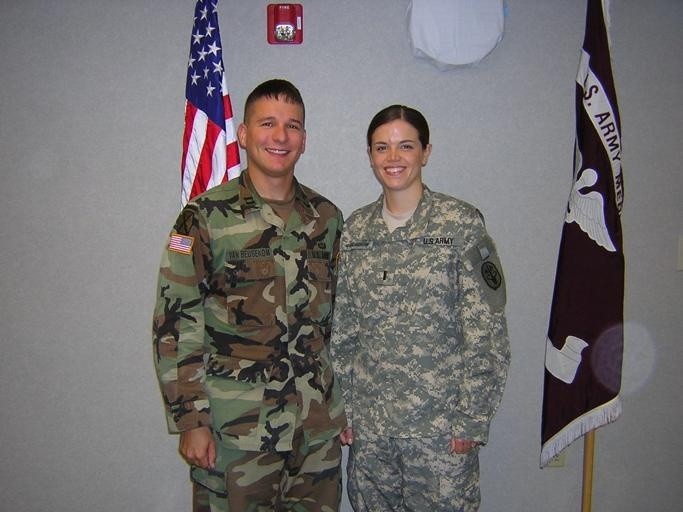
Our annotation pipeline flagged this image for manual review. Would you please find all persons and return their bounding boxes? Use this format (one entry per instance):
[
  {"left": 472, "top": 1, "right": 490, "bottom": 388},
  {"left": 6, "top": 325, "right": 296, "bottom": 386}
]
[
  {"left": 331, "top": 106, "right": 510, "bottom": 512},
  {"left": 153, "top": 79, "right": 348, "bottom": 510}
]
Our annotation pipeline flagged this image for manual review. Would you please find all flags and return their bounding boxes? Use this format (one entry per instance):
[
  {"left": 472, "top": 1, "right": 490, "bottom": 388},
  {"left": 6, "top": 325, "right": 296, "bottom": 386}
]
[
  {"left": 540, "top": 2, "right": 626, "bottom": 468},
  {"left": 180, "top": 0, "right": 240, "bottom": 207}
]
